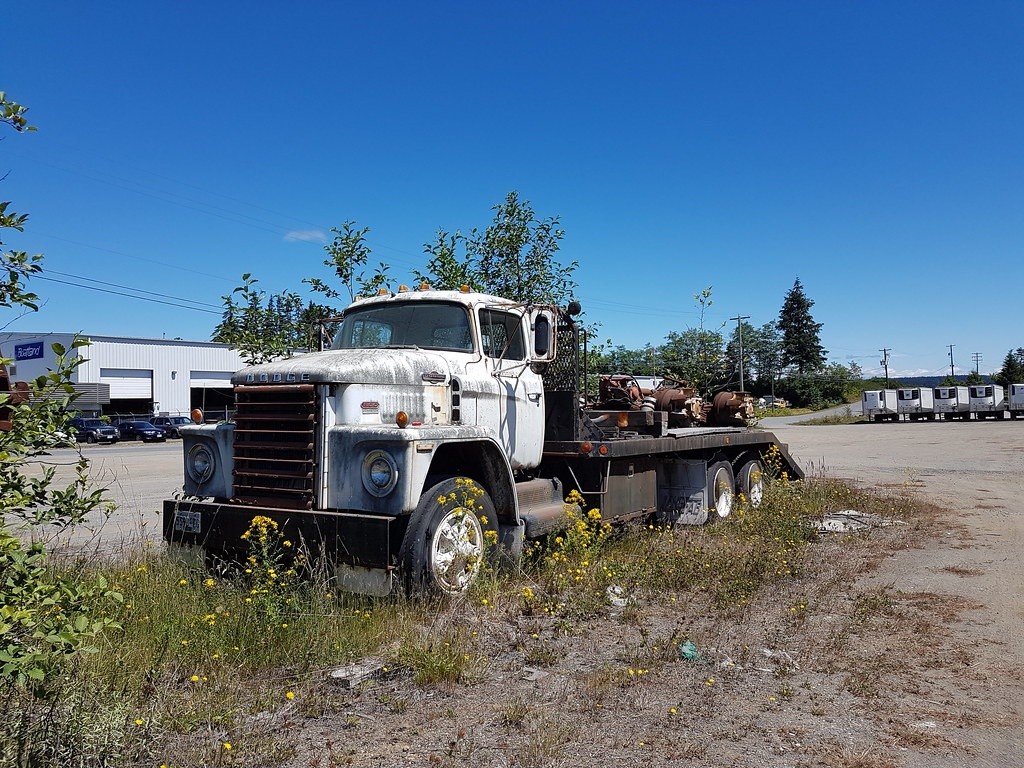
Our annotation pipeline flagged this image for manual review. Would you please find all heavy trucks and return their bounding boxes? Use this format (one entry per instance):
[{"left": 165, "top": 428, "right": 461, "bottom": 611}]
[{"left": 162, "top": 283, "right": 805, "bottom": 608}]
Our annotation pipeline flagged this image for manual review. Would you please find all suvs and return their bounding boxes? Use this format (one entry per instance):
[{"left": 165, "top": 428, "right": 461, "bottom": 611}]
[
  {"left": 65, "top": 416, "right": 121, "bottom": 444},
  {"left": 148, "top": 415, "right": 197, "bottom": 439}
]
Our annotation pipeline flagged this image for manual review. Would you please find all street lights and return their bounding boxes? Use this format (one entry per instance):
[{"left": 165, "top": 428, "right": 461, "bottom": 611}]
[
  {"left": 946, "top": 345, "right": 956, "bottom": 376},
  {"left": 771, "top": 368, "right": 778, "bottom": 413},
  {"left": 729, "top": 315, "right": 750, "bottom": 391}
]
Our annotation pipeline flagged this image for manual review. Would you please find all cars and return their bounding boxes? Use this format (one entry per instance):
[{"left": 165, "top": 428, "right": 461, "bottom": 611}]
[
  {"left": 115, "top": 421, "right": 167, "bottom": 443},
  {"left": 770, "top": 400, "right": 786, "bottom": 408}
]
